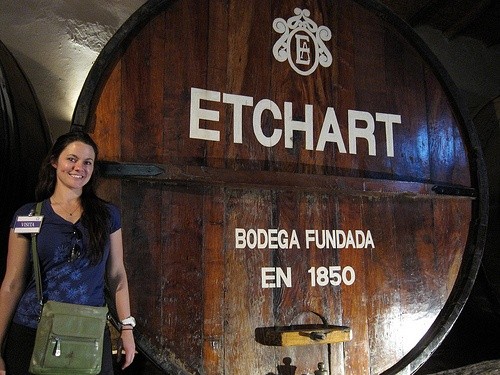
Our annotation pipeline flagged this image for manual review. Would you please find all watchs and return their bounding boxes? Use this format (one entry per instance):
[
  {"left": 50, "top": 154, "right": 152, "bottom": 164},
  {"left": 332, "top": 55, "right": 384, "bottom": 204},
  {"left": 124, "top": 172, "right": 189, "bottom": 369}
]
[{"left": 118, "top": 316, "right": 137, "bottom": 327}]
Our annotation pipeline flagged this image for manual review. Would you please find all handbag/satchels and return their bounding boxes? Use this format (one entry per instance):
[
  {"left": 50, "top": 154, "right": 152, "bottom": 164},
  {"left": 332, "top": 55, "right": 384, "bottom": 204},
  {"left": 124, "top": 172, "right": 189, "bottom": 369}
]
[{"left": 29, "top": 299, "right": 106, "bottom": 375}]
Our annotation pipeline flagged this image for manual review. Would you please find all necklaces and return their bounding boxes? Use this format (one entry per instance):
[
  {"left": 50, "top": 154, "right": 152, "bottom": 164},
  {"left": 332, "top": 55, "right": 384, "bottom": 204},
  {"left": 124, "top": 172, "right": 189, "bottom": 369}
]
[{"left": 50, "top": 196, "right": 80, "bottom": 216}]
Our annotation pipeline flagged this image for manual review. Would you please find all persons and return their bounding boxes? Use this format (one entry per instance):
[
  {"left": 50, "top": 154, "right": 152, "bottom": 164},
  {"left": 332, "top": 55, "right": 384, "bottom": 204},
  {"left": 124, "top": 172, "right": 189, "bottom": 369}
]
[{"left": 0, "top": 131, "right": 137, "bottom": 375}]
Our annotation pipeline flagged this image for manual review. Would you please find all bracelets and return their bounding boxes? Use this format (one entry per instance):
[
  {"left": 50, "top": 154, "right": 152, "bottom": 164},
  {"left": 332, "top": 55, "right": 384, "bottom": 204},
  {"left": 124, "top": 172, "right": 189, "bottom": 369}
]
[{"left": 121, "top": 328, "right": 134, "bottom": 331}]
